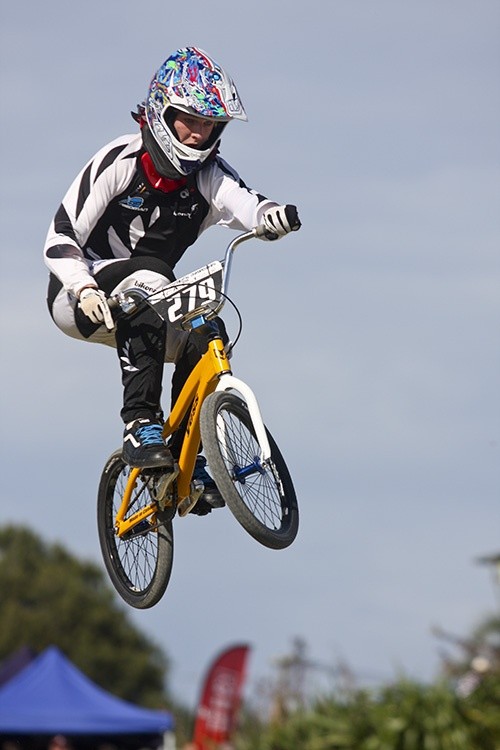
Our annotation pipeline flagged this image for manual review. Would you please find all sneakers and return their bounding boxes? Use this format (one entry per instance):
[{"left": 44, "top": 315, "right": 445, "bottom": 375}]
[
  {"left": 121, "top": 411, "right": 173, "bottom": 471},
  {"left": 179, "top": 455, "right": 225, "bottom": 508}
]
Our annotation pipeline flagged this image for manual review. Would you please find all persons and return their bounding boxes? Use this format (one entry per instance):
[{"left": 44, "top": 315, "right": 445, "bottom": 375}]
[{"left": 44, "top": 48, "right": 302, "bottom": 517}]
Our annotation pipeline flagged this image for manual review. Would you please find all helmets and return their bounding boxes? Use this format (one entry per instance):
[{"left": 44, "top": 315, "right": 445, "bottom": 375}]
[{"left": 132, "top": 47, "right": 248, "bottom": 181}]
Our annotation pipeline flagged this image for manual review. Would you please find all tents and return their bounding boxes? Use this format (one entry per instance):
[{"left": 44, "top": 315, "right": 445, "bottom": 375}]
[{"left": 0, "top": 647, "right": 182, "bottom": 750}]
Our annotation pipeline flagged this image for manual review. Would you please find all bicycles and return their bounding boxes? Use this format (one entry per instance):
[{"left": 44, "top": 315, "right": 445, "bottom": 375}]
[{"left": 95, "top": 209, "right": 302, "bottom": 611}]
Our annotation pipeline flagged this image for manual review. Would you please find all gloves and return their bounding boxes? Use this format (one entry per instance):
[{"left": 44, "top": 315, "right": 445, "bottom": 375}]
[
  {"left": 78, "top": 290, "right": 115, "bottom": 331},
  {"left": 255, "top": 202, "right": 301, "bottom": 240}
]
